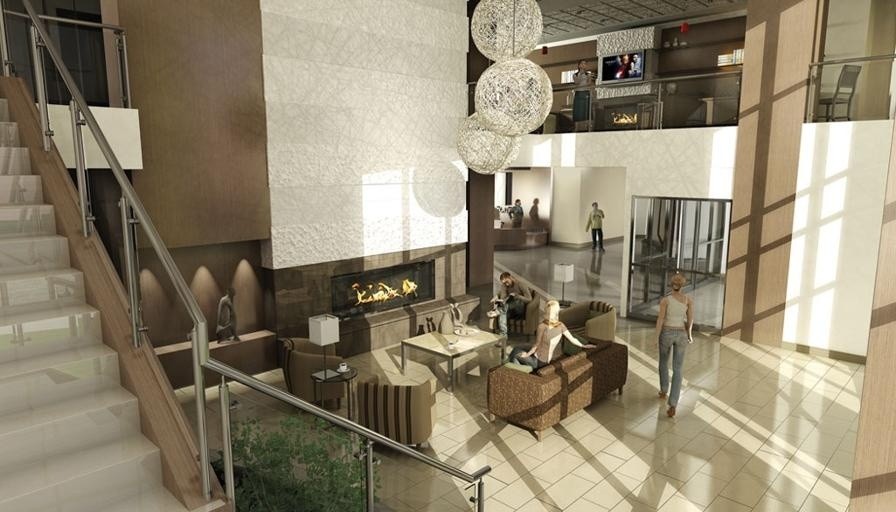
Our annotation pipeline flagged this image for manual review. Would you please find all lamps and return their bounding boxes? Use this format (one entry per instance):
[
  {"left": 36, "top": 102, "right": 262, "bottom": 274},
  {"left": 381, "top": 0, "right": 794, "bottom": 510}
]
[
  {"left": 307, "top": 313, "right": 341, "bottom": 381},
  {"left": 454, "top": 1, "right": 554, "bottom": 177},
  {"left": 554, "top": 262, "right": 574, "bottom": 306}
]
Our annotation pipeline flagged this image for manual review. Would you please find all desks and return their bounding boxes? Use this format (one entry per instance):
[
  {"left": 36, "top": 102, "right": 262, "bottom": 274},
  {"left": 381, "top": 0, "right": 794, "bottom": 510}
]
[
  {"left": 310, "top": 364, "right": 359, "bottom": 421},
  {"left": 546, "top": 298, "right": 579, "bottom": 309}
]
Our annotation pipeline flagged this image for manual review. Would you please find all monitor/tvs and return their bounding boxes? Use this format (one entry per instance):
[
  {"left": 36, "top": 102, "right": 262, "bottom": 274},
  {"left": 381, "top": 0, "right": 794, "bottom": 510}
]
[{"left": 601, "top": 50, "right": 646, "bottom": 84}]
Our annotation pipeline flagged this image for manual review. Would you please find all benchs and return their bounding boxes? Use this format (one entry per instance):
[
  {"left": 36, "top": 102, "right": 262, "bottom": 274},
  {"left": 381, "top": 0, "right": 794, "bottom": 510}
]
[{"left": 493, "top": 205, "right": 550, "bottom": 252}]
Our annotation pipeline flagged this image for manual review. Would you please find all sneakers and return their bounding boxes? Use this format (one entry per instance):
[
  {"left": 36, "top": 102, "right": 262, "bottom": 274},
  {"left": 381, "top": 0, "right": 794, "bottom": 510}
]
[
  {"left": 591, "top": 245, "right": 605, "bottom": 253},
  {"left": 487, "top": 308, "right": 501, "bottom": 318},
  {"left": 495, "top": 340, "right": 503, "bottom": 347}
]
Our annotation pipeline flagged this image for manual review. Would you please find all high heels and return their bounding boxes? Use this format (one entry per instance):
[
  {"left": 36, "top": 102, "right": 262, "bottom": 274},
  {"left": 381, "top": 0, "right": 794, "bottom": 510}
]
[
  {"left": 658, "top": 389, "right": 667, "bottom": 399},
  {"left": 667, "top": 407, "right": 676, "bottom": 418}
]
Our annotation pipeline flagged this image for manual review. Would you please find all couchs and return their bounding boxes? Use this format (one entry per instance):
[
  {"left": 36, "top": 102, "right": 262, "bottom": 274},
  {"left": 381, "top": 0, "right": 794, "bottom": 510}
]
[{"left": 482, "top": 333, "right": 629, "bottom": 443}]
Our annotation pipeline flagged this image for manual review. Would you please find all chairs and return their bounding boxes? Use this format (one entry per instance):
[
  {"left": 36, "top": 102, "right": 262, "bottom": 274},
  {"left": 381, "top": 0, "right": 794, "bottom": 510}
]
[
  {"left": 489, "top": 286, "right": 541, "bottom": 343},
  {"left": 354, "top": 373, "right": 434, "bottom": 453},
  {"left": 558, "top": 299, "right": 618, "bottom": 359},
  {"left": 818, "top": 63, "right": 863, "bottom": 123},
  {"left": 282, "top": 336, "right": 346, "bottom": 411}
]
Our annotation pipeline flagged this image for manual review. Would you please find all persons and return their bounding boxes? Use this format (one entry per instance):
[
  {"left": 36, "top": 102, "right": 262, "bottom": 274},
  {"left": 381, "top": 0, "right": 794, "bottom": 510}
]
[
  {"left": 487, "top": 273, "right": 532, "bottom": 341},
  {"left": 653, "top": 275, "right": 693, "bottom": 417},
  {"left": 216, "top": 288, "right": 240, "bottom": 343},
  {"left": 615, "top": 53, "right": 641, "bottom": 79},
  {"left": 503, "top": 299, "right": 596, "bottom": 371},
  {"left": 573, "top": 59, "right": 593, "bottom": 131},
  {"left": 509, "top": 200, "right": 523, "bottom": 228},
  {"left": 586, "top": 203, "right": 605, "bottom": 251}
]
